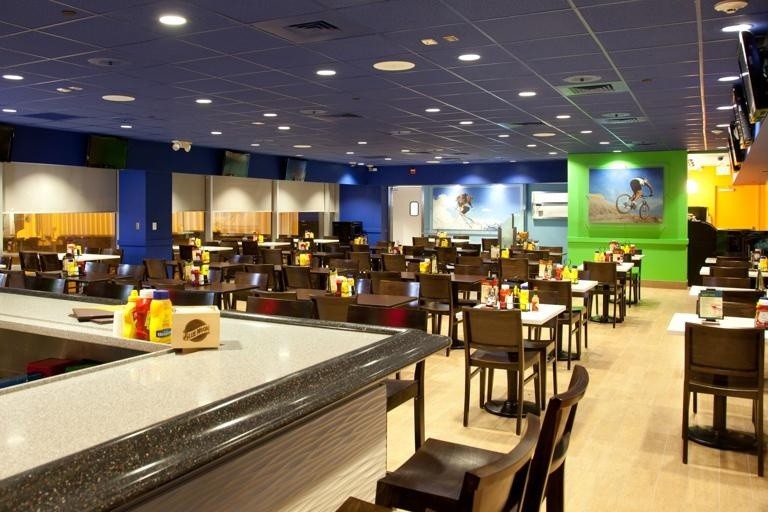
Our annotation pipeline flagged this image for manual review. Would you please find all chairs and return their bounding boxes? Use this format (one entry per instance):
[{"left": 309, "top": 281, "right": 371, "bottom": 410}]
[
  {"left": 353, "top": 279, "right": 371, "bottom": 294},
  {"left": 488, "top": 289, "right": 558, "bottom": 410},
  {"left": 329, "top": 258, "right": 359, "bottom": 278},
  {"left": 629, "top": 249, "right": 642, "bottom": 300},
  {"left": 69, "top": 262, "right": 109, "bottom": 292},
  {"left": 207, "top": 241, "right": 218, "bottom": 254},
  {"left": 143, "top": 258, "right": 169, "bottom": 281},
  {"left": 378, "top": 280, "right": 420, "bottom": 310},
  {"left": 249, "top": 288, "right": 297, "bottom": 300},
  {"left": 244, "top": 263, "right": 278, "bottom": 291},
  {"left": 527, "top": 279, "right": 581, "bottom": 370},
  {"left": 0, "top": 270, "right": 33, "bottom": 289},
  {"left": 349, "top": 251, "right": 371, "bottom": 269},
  {"left": 349, "top": 244, "right": 369, "bottom": 252},
  {"left": 245, "top": 295, "right": 313, "bottom": 320},
  {"left": 499, "top": 257, "right": 529, "bottom": 292},
  {"left": 693, "top": 299, "right": 758, "bottom": 421},
  {"left": 335, "top": 412, "right": 541, "bottom": 512},
  {"left": 539, "top": 246, "right": 562, "bottom": 264},
  {"left": 381, "top": 253, "right": 407, "bottom": 272},
  {"left": 550, "top": 271, "right": 590, "bottom": 349},
  {"left": 435, "top": 237, "right": 452, "bottom": 248},
  {"left": 325, "top": 242, "right": 344, "bottom": 252},
  {"left": 702, "top": 290, "right": 764, "bottom": 304},
  {"left": 376, "top": 364, "right": 589, "bottom": 512},
  {"left": 78, "top": 246, "right": 101, "bottom": 263},
  {"left": 462, "top": 306, "right": 541, "bottom": 436},
  {"left": 414, "top": 270, "right": 462, "bottom": 357},
  {"left": 347, "top": 304, "right": 428, "bottom": 452},
  {"left": 376, "top": 241, "right": 395, "bottom": 254},
  {"left": 435, "top": 247, "right": 456, "bottom": 274},
  {"left": 596, "top": 256, "right": 627, "bottom": 321},
  {"left": 86, "top": 284, "right": 134, "bottom": 300},
  {"left": 702, "top": 276, "right": 751, "bottom": 289},
  {"left": 526, "top": 250, "right": 549, "bottom": 263},
  {"left": 482, "top": 238, "right": 498, "bottom": 264},
  {"left": 403, "top": 245, "right": 423, "bottom": 272},
  {"left": 228, "top": 255, "right": 253, "bottom": 278},
  {"left": 242, "top": 241, "right": 261, "bottom": 262},
  {"left": 413, "top": 237, "right": 428, "bottom": 247},
  {"left": 281, "top": 264, "right": 312, "bottom": 290},
  {"left": 624, "top": 254, "right": 637, "bottom": 307},
  {"left": 682, "top": 322, "right": 765, "bottom": 478},
  {"left": 323, "top": 252, "right": 345, "bottom": 269},
  {"left": 455, "top": 256, "right": 481, "bottom": 276},
  {"left": 0, "top": 254, "right": 12, "bottom": 270},
  {"left": 230, "top": 271, "right": 269, "bottom": 309},
  {"left": 99, "top": 249, "right": 113, "bottom": 272},
  {"left": 116, "top": 264, "right": 144, "bottom": 290},
  {"left": 709, "top": 266, "right": 748, "bottom": 278},
  {"left": 462, "top": 243, "right": 481, "bottom": 256},
  {"left": 715, "top": 256, "right": 747, "bottom": 267},
  {"left": 311, "top": 295, "right": 352, "bottom": 322},
  {"left": 40, "top": 253, "right": 61, "bottom": 271},
  {"left": 371, "top": 271, "right": 401, "bottom": 293},
  {"left": 513, "top": 252, "right": 534, "bottom": 261},
  {"left": 261, "top": 248, "right": 283, "bottom": 264},
  {"left": 178, "top": 263, "right": 186, "bottom": 281},
  {"left": 33, "top": 276, "right": 67, "bottom": 293},
  {"left": 220, "top": 241, "right": 238, "bottom": 262},
  {"left": 19, "top": 251, "right": 40, "bottom": 271},
  {"left": 179, "top": 244, "right": 192, "bottom": 262},
  {"left": 583, "top": 261, "right": 623, "bottom": 329}
]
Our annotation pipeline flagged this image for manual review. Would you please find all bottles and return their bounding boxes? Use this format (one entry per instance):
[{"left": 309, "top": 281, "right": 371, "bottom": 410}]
[
  {"left": 191, "top": 267, "right": 199, "bottom": 286},
  {"left": 571, "top": 265, "right": 578, "bottom": 283},
  {"left": 594, "top": 251, "right": 599, "bottom": 262},
  {"left": 347, "top": 274, "right": 354, "bottom": 286},
  {"left": 500, "top": 284, "right": 510, "bottom": 308},
  {"left": 562, "top": 266, "right": 570, "bottom": 280},
  {"left": 543, "top": 264, "right": 548, "bottom": 280},
  {"left": 621, "top": 245, "right": 625, "bottom": 250},
  {"left": 335, "top": 276, "right": 343, "bottom": 296},
  {"left": 520, "top": 286, "right": 529, "bottom": 309},
  {"left": 204, "top": 250, "right": 209, "bottom": 263},
  {"left": 625, "top": 244, "right": 630, "bottom": 253},
  {"left": 532, "top": 287, "right": 539, "bottom": 311},
  {"left": 68, "top": 259, "right": 74, "bottom": 276},
  {"left": 631, "top": 245, "right": 635, "bottom": 255},
  {"left": 556, "top": 265, "right": 563, "bottom": 280},
  {"left": 605, "top": 252, "right": 611, "bottom": 262},
  {"left": 599, "top": 252, "right": 605, "bottom": 262}
]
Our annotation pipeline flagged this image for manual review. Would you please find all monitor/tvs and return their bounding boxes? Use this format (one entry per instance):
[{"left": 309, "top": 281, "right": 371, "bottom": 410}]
[
  {"left": 729, "top": 82, "right": 753, "bottom": 149},
  {"left": 735, "top": 28, "right": 768, "bottom": 123},
  {"left": 725, "top": 123, "right": 743, "bottom": 168},
  {"left": 726, "top": 144, "right": 740, "bottom": 172}
]
